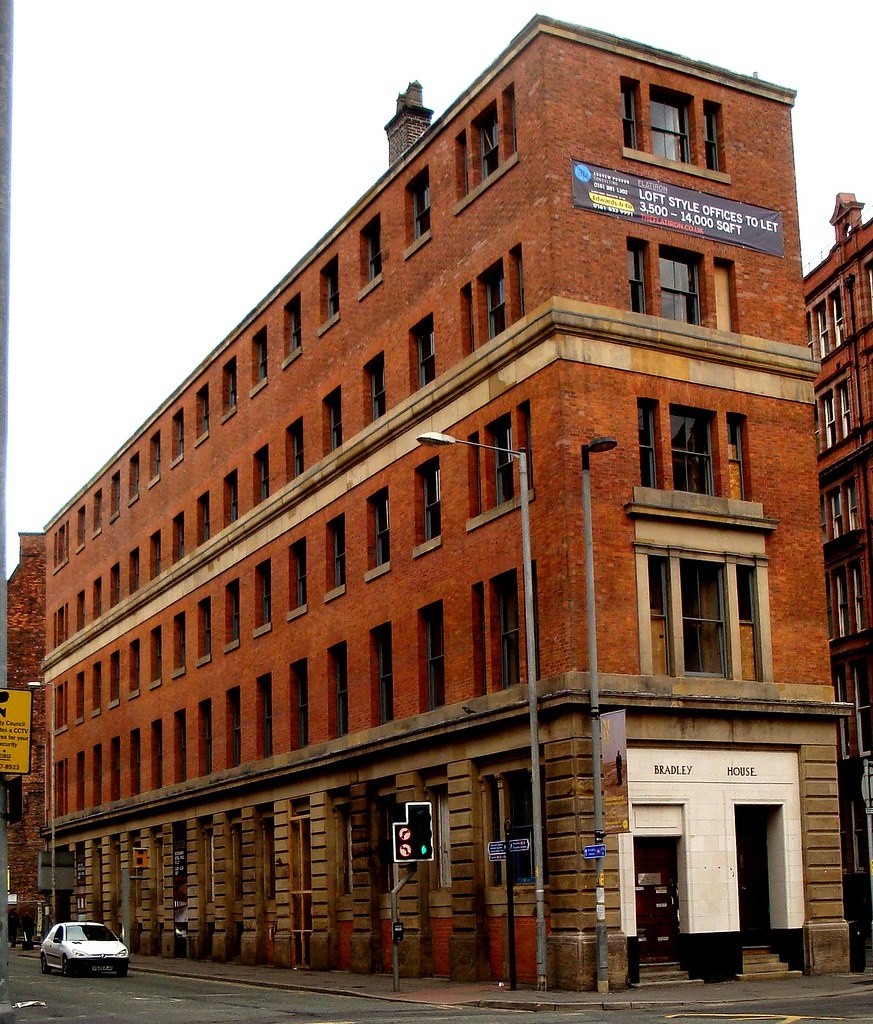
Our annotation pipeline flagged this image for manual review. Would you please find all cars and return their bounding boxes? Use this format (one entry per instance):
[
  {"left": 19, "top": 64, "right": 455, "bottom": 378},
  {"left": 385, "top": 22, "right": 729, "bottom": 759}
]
[{"left": 39, "top": 920, "right": 130, "bottom": 977}]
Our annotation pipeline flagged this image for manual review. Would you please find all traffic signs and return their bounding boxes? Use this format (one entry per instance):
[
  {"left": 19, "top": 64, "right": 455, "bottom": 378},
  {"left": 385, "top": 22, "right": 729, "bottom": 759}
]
[{"left": 487, "top": 838, "right": 529, "bottom": 861}]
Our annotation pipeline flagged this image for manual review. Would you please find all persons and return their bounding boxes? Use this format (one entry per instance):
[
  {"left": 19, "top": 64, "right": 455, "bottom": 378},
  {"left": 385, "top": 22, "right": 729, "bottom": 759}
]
[
  {"left": 22, "top": 912, "right": 35, "bottom": 950},
  {"left": 8, "top": 908, "right": 21, "bottom": 948}
]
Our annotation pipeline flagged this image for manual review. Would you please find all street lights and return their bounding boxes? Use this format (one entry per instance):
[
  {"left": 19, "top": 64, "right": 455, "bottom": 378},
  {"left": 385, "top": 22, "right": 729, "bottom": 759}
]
[
  {"left": 415, "top": 429, "right": 552, "bottom": 993},
  {"left": 580, "top": 436, "right": 620, "bottom": 994},
  {"left": 26, "top": 680, "right": 58, "bottom": 928}
]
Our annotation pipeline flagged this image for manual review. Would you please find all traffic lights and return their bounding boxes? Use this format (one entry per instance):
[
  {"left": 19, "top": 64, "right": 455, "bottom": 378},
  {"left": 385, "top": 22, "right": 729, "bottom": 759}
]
[{"left": 393, "top": 802, "right": 436, "bottom": 863}]
